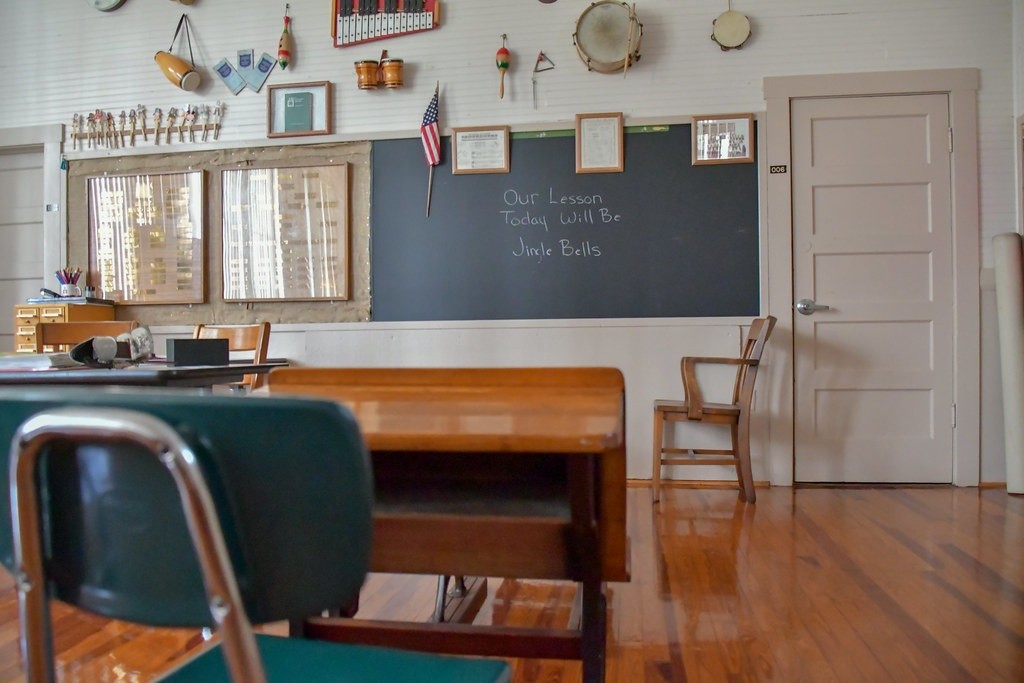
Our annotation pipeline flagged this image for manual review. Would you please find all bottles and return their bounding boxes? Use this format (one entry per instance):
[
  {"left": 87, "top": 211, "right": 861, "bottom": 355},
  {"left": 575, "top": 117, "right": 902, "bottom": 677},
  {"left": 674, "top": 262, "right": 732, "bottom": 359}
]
[{"left": 85, "top": 286, "right": 96, "bottom": 298}]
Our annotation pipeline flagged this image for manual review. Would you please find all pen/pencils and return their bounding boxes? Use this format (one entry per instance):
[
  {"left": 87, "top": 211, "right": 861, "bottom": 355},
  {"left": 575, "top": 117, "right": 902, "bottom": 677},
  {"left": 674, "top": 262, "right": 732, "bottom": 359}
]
[{"left": 54, "top": 266, "right": 83, "bottom": 284}]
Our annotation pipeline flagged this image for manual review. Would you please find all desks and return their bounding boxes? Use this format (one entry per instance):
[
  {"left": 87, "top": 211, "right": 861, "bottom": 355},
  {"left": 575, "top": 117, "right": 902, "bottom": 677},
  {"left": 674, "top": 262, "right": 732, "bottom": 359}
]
[
  {"left": 248, "top": 365, "right": 629, "bottom": 683},
  {"left": 0, "top": 364, "right": 291, "bottom": 395}
]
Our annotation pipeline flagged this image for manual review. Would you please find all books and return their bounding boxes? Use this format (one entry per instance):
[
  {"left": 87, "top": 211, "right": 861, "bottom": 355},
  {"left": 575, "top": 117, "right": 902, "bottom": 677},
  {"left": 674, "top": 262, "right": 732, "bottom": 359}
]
[{"left": 285, "top": 92, "right": 312, "bottom": 132}]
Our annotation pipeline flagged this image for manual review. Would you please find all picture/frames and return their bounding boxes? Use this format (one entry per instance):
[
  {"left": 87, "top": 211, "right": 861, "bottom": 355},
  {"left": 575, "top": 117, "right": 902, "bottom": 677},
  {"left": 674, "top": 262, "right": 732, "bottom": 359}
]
[
  {"left": 221, "top": 161, "right": 352, "bottom": 303},
  {"left": 691, "top": 114, "right": 754, "bottom": 165},
  {"left": 84, "top": 169, "right": 207, "bottom": 305}
]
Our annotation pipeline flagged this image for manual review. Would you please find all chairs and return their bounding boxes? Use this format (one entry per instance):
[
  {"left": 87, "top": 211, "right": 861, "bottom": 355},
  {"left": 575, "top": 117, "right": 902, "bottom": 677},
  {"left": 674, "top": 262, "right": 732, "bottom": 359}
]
[
  {"left": 652, "top": 315, "right": 780, "bottom": 504},
  {"left": 0, "top": 383, "right": 513, "bottom": 683},
  {"left": 193, "top": 321, "right": 270, "bottom": 363},
  {"left": 36, "top": 319, "right": 139, "bottom": 353}
]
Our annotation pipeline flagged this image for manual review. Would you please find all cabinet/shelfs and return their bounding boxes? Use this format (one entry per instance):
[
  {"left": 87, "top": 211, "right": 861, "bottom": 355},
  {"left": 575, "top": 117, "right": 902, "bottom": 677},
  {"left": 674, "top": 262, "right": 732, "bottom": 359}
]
[{"left": 13, "top": 304, "right": 115, "bottom": 352}]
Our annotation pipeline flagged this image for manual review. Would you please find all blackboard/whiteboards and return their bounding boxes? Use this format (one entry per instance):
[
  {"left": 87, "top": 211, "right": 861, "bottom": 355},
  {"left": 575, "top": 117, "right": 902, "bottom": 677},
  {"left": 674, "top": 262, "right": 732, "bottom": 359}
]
[{"left": 369, "top": 112, "right": 766, "bottom": 330}]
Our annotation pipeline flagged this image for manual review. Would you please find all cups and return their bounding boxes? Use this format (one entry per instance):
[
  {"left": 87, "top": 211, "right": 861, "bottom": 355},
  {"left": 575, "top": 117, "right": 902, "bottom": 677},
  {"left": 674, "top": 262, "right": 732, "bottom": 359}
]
[{"left": 60, "top": 284, "right": 82, "bottom": 297}]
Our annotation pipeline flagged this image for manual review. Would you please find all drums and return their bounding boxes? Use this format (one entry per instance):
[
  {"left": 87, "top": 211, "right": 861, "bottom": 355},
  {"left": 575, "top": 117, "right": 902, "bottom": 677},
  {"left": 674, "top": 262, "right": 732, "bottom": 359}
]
[
  {"left": 154, "top": 50, "right": 202, "bottom": 91},
  {"left": 353, "top": 58, "right": 404, "bottom": 90},
  {"left": 713, "top": 11, "right": 751, "bottom": 47},
  {"left": 572, "top": 0, "right": 642, "bottom": 74}
]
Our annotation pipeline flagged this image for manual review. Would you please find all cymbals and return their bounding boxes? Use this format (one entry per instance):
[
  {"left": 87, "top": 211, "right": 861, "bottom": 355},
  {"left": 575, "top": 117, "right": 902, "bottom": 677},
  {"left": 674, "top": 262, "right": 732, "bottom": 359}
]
[
  {"left": 720, "top": 45, "right": 730, "bottom": 51},
  {"left": 712, "top": 19, "right": 717, "bottom": 26},
  {"left": 736, "top": 43, "right": 744, "bottom": 50},
  {"left": 710, "top": 33, "right": 716, "bottom": 41},
  {"left": 748, "top": 31, "right": 752, "bottom": 37}
]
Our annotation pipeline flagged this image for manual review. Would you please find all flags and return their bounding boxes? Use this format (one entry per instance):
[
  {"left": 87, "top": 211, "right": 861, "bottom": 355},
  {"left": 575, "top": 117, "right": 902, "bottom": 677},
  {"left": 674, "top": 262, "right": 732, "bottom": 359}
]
[{"left": 421, "top": 89, "right": 441, "bottom": 166}]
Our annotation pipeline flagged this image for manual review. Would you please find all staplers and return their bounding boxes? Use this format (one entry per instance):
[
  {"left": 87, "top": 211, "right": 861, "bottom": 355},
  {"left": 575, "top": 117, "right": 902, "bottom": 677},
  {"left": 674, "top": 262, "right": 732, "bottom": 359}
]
[{"left": 40, "top": 288, "right": 62, "bottom": 298}]
